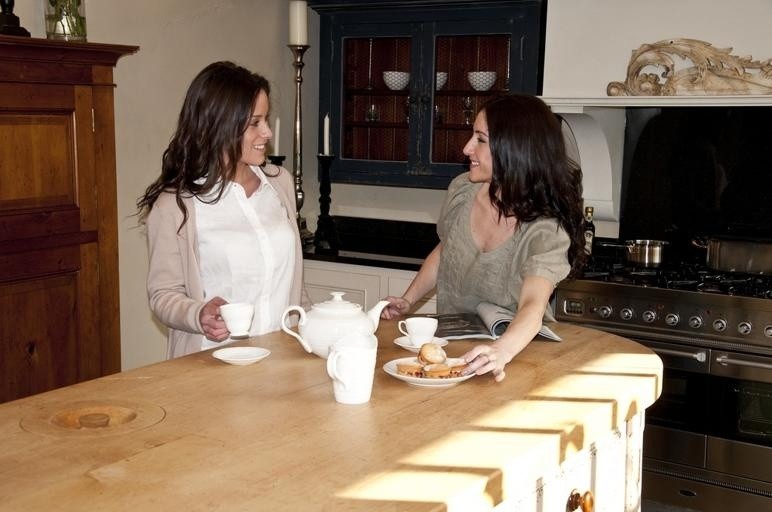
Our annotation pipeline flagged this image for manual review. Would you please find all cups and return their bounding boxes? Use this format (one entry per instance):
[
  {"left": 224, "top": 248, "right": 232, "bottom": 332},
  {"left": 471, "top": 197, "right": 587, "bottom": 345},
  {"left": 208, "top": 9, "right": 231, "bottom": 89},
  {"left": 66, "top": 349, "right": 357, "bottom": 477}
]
[
  {"left": 326, "top": 330, "right": 378, "bottom": 404},
  {"left": 398, "top": 317, "right": 438, "bottom": 343},
  {"left": 219, "top": 303, "right": 255, "bottom": 337}
]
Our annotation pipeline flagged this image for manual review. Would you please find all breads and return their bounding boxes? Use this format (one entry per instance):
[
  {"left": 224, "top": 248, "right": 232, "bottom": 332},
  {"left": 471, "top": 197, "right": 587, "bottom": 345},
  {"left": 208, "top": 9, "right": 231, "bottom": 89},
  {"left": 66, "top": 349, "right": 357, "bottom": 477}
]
[{"left": 397, "top": 342, "right": 470, "bottom": 378}]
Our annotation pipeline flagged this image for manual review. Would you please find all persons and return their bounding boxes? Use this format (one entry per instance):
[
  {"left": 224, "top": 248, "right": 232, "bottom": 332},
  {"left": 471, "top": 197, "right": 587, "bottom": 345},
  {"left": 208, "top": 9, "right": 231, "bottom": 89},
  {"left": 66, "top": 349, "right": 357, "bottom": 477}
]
[
  {"left": 127, "top": 61, "right": 303, "bottom": 360},
  {"left": 380, "top": 94, "right": 588, "bottom": 383}
]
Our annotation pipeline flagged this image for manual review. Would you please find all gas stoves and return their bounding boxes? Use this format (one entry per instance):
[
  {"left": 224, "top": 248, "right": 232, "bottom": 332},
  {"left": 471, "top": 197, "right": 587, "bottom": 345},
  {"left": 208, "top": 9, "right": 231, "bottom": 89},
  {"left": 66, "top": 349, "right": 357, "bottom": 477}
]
[{"left": 555, "top": 260, "right": 772, "bottom": 347}]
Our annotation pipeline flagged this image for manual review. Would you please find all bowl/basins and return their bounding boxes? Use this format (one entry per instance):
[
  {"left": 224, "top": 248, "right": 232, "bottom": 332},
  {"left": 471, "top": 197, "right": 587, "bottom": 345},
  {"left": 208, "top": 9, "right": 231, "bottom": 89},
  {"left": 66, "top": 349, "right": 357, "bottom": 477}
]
[
  {"left": 466, "top": 71, "right": 497, "bottom": 91},
  {"left": 382, "top": 70, "right": 410, "bottom": 90},
  {"left": 436, "top": 71, "right": 447, "bottom": 90}
]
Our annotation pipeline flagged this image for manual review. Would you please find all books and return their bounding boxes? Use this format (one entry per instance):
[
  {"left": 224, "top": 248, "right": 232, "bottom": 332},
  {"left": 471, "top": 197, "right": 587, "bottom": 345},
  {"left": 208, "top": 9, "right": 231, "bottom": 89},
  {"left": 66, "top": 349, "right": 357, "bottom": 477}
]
[{"left": 427, "top": 302, "right": 562, "bottom": 342}]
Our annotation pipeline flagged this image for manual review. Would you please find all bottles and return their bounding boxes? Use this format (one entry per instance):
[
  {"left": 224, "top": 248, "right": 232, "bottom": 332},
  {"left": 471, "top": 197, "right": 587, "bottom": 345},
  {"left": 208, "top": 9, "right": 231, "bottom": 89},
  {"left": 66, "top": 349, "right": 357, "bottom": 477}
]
[{"left": 581, "top": 207, "right": 595, "bottom": 267}]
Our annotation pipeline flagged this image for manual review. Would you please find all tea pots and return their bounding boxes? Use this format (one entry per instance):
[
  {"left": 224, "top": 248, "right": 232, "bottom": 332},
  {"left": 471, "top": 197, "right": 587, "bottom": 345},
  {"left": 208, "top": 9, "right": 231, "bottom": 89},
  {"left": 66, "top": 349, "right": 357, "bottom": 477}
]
[{"left": 283, "top": 290, "right": 388, "bottom": 359}]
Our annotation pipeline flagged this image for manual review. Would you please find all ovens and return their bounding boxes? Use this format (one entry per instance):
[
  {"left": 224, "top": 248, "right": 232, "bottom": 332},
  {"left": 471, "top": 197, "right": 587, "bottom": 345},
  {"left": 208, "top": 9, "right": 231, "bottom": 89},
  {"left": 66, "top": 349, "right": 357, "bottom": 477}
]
[{"left": 628, "top": 339, "right": 771, "bottom": 512}]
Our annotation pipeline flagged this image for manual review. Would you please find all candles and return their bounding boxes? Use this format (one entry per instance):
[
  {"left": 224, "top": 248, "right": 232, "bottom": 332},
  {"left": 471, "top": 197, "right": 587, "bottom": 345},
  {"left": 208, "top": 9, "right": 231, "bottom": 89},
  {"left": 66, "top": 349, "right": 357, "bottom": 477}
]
[
  {"left": 324, "top": 113, "right": 330, "bottom": 155},
  {"left": 288, "top": 0, "right": 305, "bottom": 45},
  {"left": 274, "top": 117, "right": 280, "bottom": 155}
]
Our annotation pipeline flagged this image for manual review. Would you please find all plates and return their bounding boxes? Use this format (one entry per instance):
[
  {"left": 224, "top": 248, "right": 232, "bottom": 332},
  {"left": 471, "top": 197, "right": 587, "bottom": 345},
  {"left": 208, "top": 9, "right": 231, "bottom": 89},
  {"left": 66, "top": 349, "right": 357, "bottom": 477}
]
[
  {"left": 393, "top": 337, "right": 450, "bottom": 353},
  {"left": 212, "top": 347, "right": 271, "bottom": 365},
  {"left": 382, "top": 357, "right": 476, "bottom": 387}
]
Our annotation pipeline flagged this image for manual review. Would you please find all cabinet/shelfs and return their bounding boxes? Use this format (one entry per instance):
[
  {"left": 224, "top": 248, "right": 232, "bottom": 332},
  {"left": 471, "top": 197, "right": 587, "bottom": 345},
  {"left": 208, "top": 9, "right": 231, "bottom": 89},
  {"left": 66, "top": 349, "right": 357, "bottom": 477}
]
[
  {"left": 298, "top": 262, "right": 436, "bottom": 316},
  {"left": 307, "top": 0, "right": 547, "bottom": 192},
  {"left": 0, "top": 35, "right": 139, "bottom": 404},
  {"left": 504, "top": 421, "right": 626, "bottom": 512}
]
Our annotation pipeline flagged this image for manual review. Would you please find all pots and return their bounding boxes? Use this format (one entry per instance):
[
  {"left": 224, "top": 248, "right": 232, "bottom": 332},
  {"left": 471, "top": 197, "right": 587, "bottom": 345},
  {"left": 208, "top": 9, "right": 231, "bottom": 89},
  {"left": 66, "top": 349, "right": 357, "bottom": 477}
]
[
  {"left": 598, "top": 238, "right": 671, "bottom": 266},
  {"left": 691, "top": 238, "right": 772, "bottom": 273}
]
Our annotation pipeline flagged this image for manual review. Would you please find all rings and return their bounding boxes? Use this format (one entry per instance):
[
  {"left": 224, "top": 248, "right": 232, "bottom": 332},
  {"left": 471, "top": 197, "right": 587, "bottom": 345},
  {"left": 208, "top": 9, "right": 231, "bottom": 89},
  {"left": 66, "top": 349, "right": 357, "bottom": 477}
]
[{"left": 485, "top": 355, "right": 490, "bottom": 363}]
